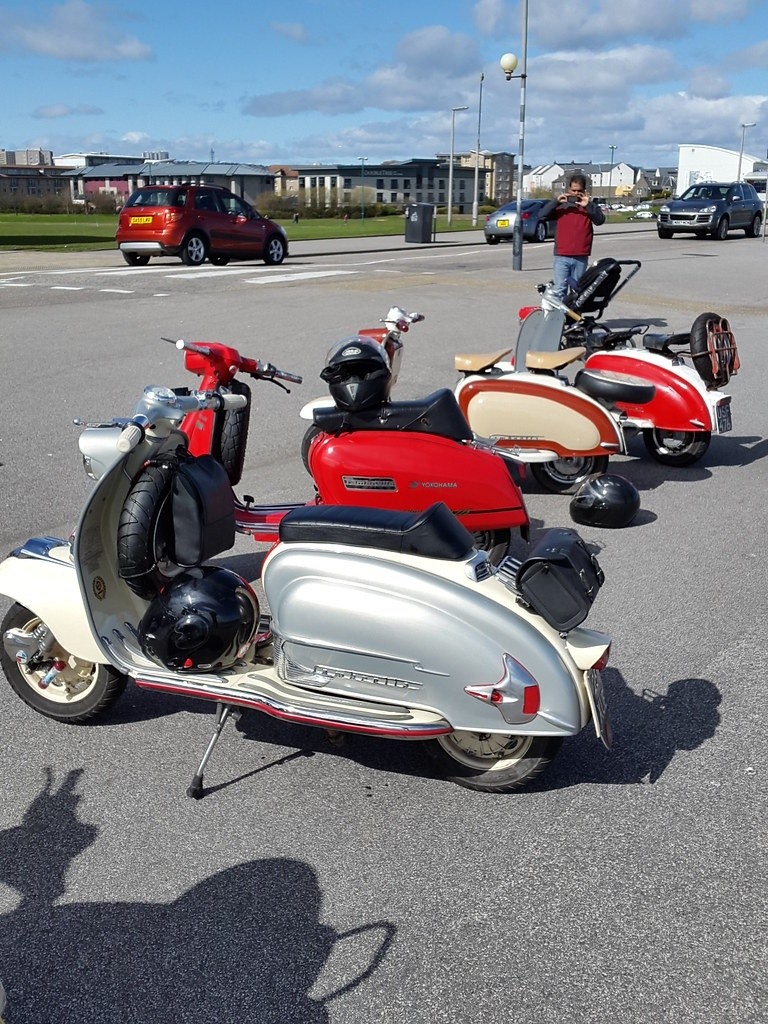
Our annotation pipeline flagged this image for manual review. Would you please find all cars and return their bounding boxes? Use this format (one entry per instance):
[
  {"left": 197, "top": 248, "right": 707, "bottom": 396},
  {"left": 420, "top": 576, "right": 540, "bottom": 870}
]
[
  {"left": 627, "top": 211, "right": 657, "bottom": 220},
  {"left": 484, "top": 199, "right": 557, "bottom": 245},
  {"left": 598, "top": 203, "right": 652, "bottom": 212}
]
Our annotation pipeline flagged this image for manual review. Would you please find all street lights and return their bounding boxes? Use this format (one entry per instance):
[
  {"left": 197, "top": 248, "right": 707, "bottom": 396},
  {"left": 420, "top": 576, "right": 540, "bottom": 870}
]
[
  {"left": 500, "top": 53, "right": 527, "bottom": 272},
  {"left": 608, "top": 145, "right": 617, "bottom": 206},
  {"left": 737, "top": 123, "right": 757, "bottom": 181},
  {"left": 448, "top": 106, "right": 469, "bottom": 225},
  {"left": 357, "top": 157, "right": 368, "bottom": 220}
]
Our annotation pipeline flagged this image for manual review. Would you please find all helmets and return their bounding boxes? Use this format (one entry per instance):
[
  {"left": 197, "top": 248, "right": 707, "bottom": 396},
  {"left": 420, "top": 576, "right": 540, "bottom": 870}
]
[
  {"left": 136, "top": 566, "right": 259, "bottom": 673},
  {"left": 320, "top": 335, "right": 394, "bottom": 410},
  {"left": 569, "top": 475, "right": 640, "bottom": 528}
]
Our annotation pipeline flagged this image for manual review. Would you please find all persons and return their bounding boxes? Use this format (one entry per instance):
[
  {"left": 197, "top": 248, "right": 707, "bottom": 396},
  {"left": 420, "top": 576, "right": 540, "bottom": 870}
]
[{"left": 537, "top": 173, "right": 605, "bottom": 299}]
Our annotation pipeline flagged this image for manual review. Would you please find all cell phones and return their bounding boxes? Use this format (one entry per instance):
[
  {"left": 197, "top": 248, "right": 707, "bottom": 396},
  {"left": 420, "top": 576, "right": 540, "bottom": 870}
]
[{"left": 567, "top": 196, "right": 580, "bottom": 202}]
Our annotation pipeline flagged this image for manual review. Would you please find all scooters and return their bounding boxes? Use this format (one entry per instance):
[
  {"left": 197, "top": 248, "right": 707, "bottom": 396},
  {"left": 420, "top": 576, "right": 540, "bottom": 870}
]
[
  {"left": 1, "top": 385, "right": 614, "bottom": 797},
  {"left": 143, "top": 339, "right": 531, "bottom": 572},
  {"left": 299, "top": 258, "right": 741, "bottom": 494}
]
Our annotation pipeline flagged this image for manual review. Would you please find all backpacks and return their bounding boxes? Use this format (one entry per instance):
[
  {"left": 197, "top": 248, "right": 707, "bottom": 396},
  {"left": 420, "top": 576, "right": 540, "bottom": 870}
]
[
  {"left": 564, "top": 258, "right": 622, "bottom": 320},
  {"left": 172, "top": 454, "right": 237, "bottom": 568}
]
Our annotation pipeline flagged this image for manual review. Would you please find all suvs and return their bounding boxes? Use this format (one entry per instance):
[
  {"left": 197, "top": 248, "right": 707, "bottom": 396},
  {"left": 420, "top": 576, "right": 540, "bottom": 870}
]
[
  {"left": 116, "top": 182, "right": 290, "bottom": 267},
  {"left": 657, "top": 181, "right": 764, "bottom": 240}
]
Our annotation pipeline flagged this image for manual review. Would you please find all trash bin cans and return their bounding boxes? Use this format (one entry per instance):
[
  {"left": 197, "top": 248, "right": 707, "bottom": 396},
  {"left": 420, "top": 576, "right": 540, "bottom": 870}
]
[{"left": 404, "top": 203, "right": 435, "bottom": 243}]
